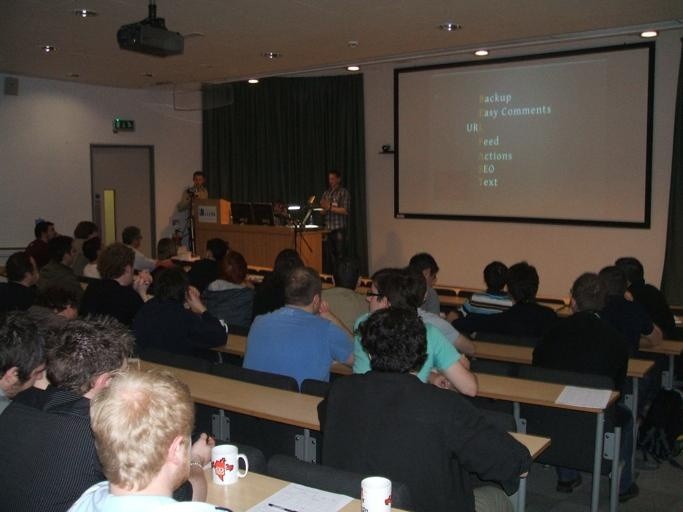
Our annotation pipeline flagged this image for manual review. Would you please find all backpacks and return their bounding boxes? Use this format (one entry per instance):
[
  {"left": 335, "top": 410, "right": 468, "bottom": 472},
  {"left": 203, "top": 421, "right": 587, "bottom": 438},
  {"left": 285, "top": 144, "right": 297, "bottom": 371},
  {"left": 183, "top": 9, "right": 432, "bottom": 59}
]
[{"left": 637, "top": 387, "right": 683, "bottom": 469}]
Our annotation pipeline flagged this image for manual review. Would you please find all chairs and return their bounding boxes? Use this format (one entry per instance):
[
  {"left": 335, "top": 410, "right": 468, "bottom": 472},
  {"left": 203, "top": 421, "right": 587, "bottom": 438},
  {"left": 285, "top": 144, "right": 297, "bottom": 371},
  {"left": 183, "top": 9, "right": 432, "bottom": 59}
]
[
  {"left": 268, "top": 454, "right": 412, "bottom": 510},
  {"left": 214, "top": 363, "right": 299, "bottom": 393},
  {"left": 465, "top": 311, "right": 505, "bottom": 328},
  {"left": 519, "top": 366, "right": 627, "bottom": 511},
  {"left": 216, "top": 442, "right": 266, "bottom": 475},
  {"left": 301, "top": 379, "right": 332, "bottom": 398}
]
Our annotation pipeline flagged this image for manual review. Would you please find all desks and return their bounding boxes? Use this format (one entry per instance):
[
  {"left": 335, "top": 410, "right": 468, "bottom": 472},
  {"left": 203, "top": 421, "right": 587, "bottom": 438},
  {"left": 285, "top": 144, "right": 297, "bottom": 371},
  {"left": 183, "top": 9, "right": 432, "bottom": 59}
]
[
  {"left": 143, "top": 359, "right": 552, "bottom": 512},
  {"left": 210, "top": 333, "right": 655, "bottom": 483},
  {"left": 247, "top": 263, "right": 683, "bottom": 314},
  {"left": 437, "top": 295, "right": 683, "bottom": 390},
  {"left": 204, "top": 462, "right": 410, "bottom": 511}
]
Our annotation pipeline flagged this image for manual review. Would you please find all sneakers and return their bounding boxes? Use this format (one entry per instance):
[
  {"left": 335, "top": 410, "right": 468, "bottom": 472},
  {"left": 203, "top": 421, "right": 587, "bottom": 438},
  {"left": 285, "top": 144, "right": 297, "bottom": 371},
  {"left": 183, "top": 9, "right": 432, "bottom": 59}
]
[
  {"left": 556, "top": 472, "right": 582, "bottom": 493},
  {"left": 615, "top": 482, "right": 640, "bottom": 501}
]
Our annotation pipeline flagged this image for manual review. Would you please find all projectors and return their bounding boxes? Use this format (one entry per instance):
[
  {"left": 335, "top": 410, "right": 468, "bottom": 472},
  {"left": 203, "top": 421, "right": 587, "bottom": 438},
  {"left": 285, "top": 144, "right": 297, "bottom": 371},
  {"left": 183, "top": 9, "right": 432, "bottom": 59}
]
[{"left": 116, "top": 0, "right": 184, "bottom": 58}]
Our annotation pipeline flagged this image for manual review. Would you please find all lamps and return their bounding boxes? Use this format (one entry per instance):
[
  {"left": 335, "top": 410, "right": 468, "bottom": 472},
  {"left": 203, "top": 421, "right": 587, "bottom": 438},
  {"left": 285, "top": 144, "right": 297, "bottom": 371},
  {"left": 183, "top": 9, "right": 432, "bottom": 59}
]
[
  {"left": 286, "top": 205, "right": 302, "bottom": 228},
  {"left": 305, "top": 207, "right": 324, "bottom": 229}
]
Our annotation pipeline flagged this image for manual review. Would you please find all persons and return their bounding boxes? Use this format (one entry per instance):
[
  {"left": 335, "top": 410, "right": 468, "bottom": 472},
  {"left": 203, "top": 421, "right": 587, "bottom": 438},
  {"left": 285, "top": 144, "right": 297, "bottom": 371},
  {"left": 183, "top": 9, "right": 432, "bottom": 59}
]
[
  {"left": 65, "top": 365, "right": 242, "bottom": 512},
  {"left": 253, "top": 248, "right": 302, "bottom": 316},
  {"left": 447, "top": 262, "right": 513, "bottom": 324},
  {"left": 146, "top": 238, "right": 190, "bottom": 294},
  {"left": 26, "top": 287, "right": 79, "bottom": 337},
  {"left": 317, "top": 306, "right": 532, "bottom": 511},
  {"left": 353, "top": 268, "right": 481, "bottom": 401},
  {"left": 409, "top": 252, "right": 445, "bottom": 327},
  {"left": 35, "top": 238, "right": 82, "bottom": 295},
  {"left": 201, "top": 251, "right": 258, "bottom": 330},
  {"left": 319, "top": 171, "right": 356, "bottom": 281},
  {"left": 189, "top": 238, "right": 230, "bottom": 295},
  {"left": 0, "top": 315, "right": 212, "bottom": 511},
  {"left": 81, "top": 237, "right": 107, "bottom": 278},
  {"left": 615, "top": 257, "right": 677, "bottom": 341},
  {"left": 398, "top": 267, "right": 478, "bottom": 356},
  {"left": 178, "top": 172, "right": 209, "bottom": 212},
  {"left": 242, "top": 266, "right": 357, "bottom": 393},
  {"left": 25, "top": 221, "right": 61, "bottom": 271},
  {"left": 599, "top": 266, "right": 661, "bottom": 423},
  {"left": 79, "top": 243, "right": 154, "bottom": 331},
  {"left": 0, "top": 315, "right": 50, "bottom": 416},
  {"left": 121, "top": 226, "right": 160, "bottom": 272},
  {"left": 532, "top": 273, "right": 642, "bottom": 504},
  {"left": 477, "top": 261, "right": 559, "bottom": 341},
  {"left": 130, "top": 265, "right": 228, "bottom": 373},
  {"left": 2, "top": 251, "right": 40, "bottom": 311},
  {"left": 318, "top": 260, "right": 370, "bottom": 333},
  {"left": 272, "top": 199, "right": 291, "bottom": 226},
  {"left": 71, "top": 222, "right": 97, "bottom": 272}
]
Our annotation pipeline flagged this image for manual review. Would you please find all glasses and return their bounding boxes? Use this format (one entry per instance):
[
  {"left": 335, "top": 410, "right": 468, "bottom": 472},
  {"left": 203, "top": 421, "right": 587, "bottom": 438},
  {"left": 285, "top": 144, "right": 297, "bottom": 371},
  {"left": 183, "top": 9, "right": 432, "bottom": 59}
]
[
  {"left": 366, "top": 288, "right": 383, "bottom": 297},
  {"left": 137, "top": 236, "right": 143, "bottom": 240}
]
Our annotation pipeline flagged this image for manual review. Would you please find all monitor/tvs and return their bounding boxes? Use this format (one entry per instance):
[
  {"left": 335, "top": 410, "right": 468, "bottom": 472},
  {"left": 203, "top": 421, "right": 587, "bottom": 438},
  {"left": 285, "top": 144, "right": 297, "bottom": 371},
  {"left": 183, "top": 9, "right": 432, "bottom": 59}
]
[
  {"left": 231, "top": 201, "right": 252, "bottom": 224},
  {"left": 253, "top": 202, "right": 274, "bottom": 225}
]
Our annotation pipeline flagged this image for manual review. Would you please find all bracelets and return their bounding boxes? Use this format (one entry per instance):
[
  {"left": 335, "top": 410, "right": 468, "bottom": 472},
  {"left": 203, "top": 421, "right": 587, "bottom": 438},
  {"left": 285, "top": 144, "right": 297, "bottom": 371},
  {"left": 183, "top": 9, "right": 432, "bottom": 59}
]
[
  {"left": 190, "top": 457, "right": 205, "bottom": 470},
  {"left": 328, "top": 206, "right": 333, "bottom": 211}
]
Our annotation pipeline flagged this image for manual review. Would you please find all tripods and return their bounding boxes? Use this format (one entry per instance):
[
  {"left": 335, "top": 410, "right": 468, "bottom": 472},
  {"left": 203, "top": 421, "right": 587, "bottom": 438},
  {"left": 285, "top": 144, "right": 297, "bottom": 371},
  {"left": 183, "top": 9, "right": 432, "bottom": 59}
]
[{"left": 177, "top": 192, "right": 195, "bottom": 256}]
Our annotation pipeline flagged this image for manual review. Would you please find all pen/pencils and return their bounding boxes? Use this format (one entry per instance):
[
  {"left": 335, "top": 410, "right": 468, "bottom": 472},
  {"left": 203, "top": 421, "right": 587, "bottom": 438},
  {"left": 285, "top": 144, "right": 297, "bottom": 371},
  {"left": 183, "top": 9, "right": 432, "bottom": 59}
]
[{"left": 268, "top": 503, "right": 297, "bottom": 512}]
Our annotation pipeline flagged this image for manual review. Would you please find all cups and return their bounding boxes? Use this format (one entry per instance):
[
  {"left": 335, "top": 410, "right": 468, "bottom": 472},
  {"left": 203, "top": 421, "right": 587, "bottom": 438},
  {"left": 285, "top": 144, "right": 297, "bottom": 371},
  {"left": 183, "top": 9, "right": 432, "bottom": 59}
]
[
  {"left": 219, "top": 320, "right": 228, "bottom": 335},
  {"left": 361, "top": 476, "right": 392, "bottom": 512},
  {"left": 212, "top": 444, "right": 248, "bottom": 487}
]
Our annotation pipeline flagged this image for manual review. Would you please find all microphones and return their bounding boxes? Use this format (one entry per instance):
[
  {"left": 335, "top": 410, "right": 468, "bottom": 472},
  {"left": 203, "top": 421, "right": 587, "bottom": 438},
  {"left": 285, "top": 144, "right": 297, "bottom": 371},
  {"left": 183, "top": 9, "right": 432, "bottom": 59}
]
[{"left": 307, "top": 192, "right": 315, "bottom": 205}]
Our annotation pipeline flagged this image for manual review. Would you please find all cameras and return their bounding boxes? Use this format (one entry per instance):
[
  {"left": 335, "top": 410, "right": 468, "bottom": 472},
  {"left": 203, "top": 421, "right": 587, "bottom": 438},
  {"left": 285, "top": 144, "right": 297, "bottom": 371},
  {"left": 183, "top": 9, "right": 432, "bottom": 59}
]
[{"left": 188, "top": 186, "right": 200, "bottom": 193}]
